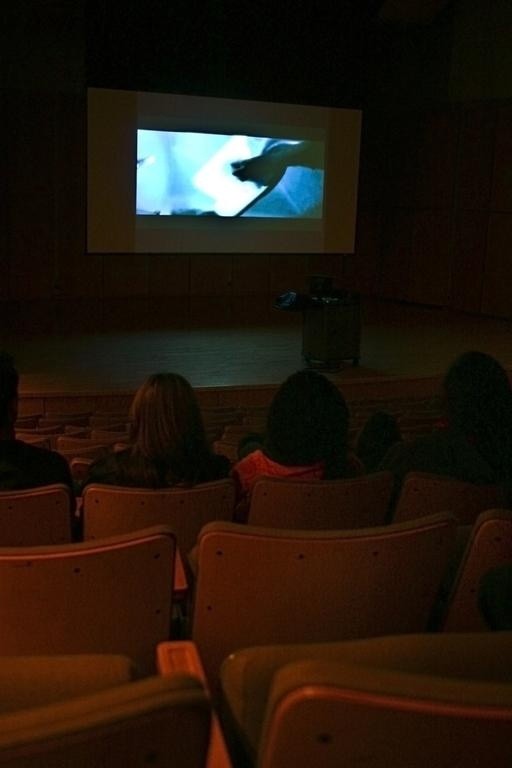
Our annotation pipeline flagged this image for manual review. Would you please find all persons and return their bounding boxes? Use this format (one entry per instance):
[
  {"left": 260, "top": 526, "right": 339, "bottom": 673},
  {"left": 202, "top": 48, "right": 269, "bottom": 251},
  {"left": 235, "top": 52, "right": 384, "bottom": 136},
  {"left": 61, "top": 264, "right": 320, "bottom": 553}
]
[
  {"left": 227, "top": 369, "right": 365, "bottom": 524},
  {"left": 77, "top": 369, "right": 232, "bottom": 490},
  {"left": 0, "top": 351, "right": 76, "bottom": 538},
  {"left": 357, "top": 351, "right": 512, "bottom": 523}
]
